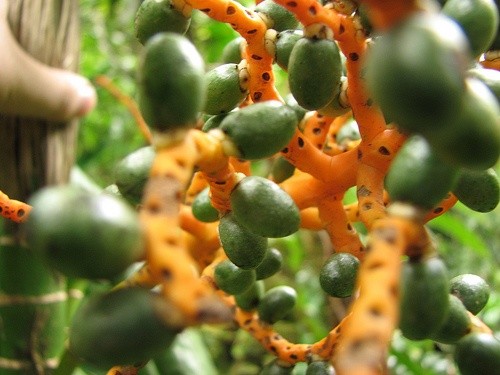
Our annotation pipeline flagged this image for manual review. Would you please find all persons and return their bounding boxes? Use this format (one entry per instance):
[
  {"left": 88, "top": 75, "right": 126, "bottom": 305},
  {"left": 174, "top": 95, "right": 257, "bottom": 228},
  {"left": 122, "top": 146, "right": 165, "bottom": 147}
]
[{"left": 0, "top": 1, "right": 96, "bottom": 121}]
